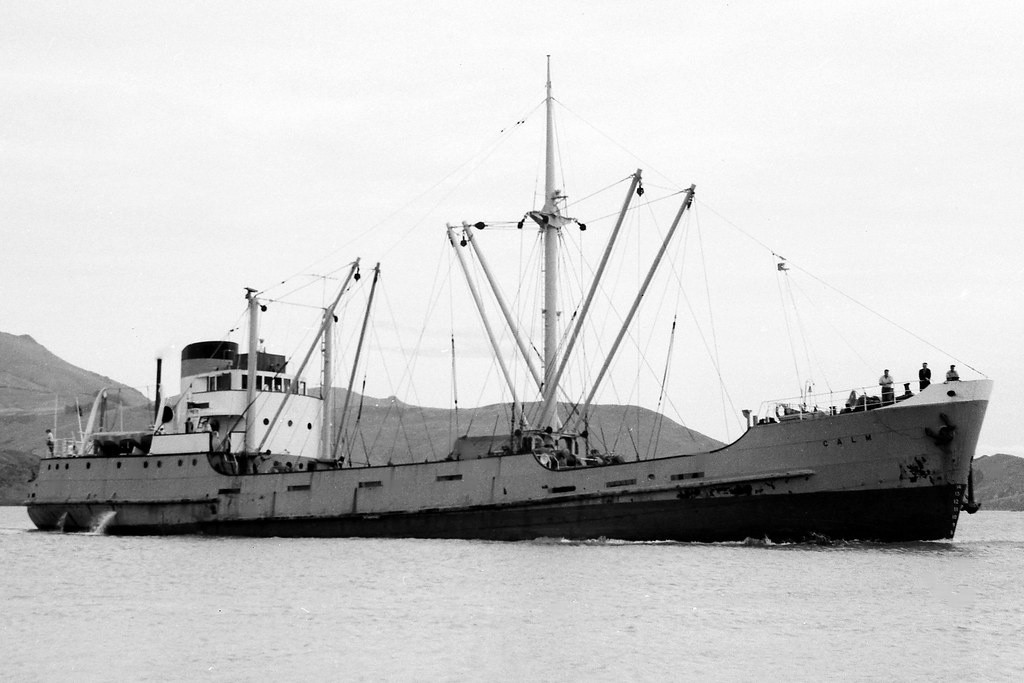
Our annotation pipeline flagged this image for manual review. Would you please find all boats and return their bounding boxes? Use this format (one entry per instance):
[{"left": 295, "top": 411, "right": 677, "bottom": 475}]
[{"left": 25, "top": 50, "right": 996, "bottom": 551}]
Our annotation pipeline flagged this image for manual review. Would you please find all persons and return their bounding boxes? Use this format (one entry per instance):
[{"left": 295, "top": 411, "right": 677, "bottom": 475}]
[
  {"left": 46, "top": 429, "right": 54, "bottom": 457},
  {"left": 185, "top": 416, "right": 212, "bottom": 433},
  {"left": 284, "top": 462, "right": 306, "bottom": 472},
  {"left": 879, "top": 370, "right": 895, "bottom": 406},
  {"left": 919, "top": 363, "right": 931, "bottom": 391},
  {"left": 946, "top": 365, "right": 959, "bottom": 381},
  {"left": 332, "top": 456, "right": 345, "bottom": 469},
  {"left": 223, "top": 448, "right": 271, "bottom": 473}
]
